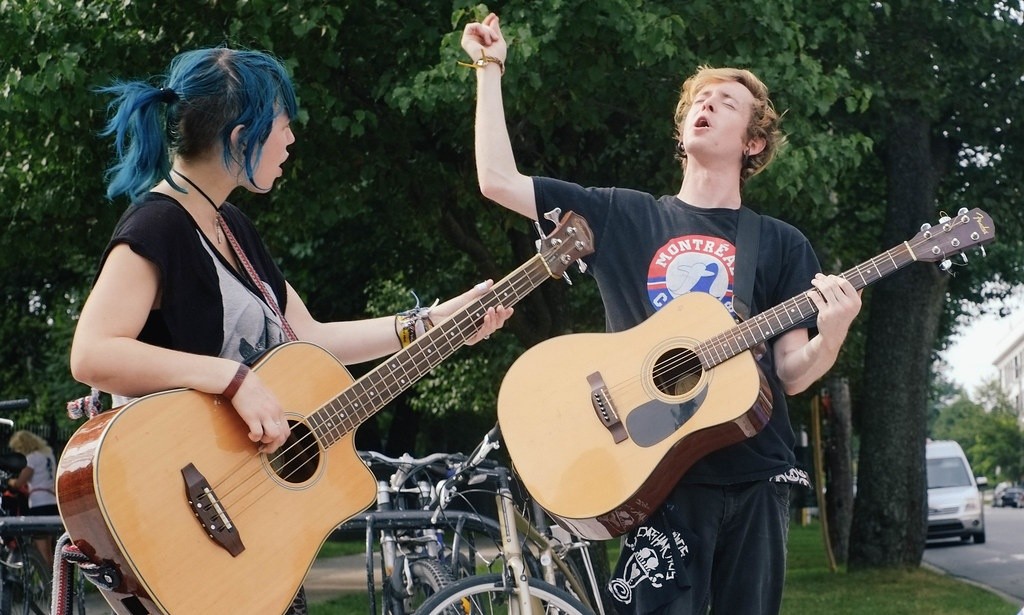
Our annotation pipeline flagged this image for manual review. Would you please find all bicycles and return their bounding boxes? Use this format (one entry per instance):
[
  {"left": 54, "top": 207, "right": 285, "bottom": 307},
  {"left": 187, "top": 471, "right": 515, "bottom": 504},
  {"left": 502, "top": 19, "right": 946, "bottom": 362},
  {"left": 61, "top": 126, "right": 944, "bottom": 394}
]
[{"left": 0, "top": 395, "right": 617, "bottom": 615}]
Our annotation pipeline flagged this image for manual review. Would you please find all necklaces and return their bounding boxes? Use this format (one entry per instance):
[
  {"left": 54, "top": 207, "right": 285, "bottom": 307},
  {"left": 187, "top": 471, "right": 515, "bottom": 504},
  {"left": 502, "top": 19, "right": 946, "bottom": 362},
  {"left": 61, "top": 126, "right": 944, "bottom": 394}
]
[{"left": 171, "top": 167, "right": 221, "bottom": 244}]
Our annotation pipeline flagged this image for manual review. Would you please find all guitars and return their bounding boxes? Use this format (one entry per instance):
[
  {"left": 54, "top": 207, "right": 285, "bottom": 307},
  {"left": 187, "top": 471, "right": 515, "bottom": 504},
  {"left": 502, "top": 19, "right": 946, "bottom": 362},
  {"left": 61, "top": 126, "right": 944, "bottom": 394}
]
[
  {"left": 55, "top": 206, "right": 595, "bottom": 615},
  {"left": 496, "top": 205, "right": 996, "bottom": 541}
]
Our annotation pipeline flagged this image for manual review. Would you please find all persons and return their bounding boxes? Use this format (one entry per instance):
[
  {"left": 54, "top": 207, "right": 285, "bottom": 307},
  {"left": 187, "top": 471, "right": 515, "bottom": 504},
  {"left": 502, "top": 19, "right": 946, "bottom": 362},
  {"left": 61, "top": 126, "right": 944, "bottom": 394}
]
[
  {"left": 459, "top": 12, "right": 864, "bottom": 615},
  {"left": 69, "top": 45, "right": 514, "bottom": 615},
  {"left": 10, "top": 431, "right": 56, "bottom": 574}
]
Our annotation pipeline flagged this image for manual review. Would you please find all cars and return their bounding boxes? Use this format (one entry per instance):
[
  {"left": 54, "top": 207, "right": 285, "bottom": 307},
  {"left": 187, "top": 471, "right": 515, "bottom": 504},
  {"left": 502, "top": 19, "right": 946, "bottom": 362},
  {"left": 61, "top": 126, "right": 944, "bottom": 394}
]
[{"left": 991, "top": 488, "right": 1024, "bottom": 508}]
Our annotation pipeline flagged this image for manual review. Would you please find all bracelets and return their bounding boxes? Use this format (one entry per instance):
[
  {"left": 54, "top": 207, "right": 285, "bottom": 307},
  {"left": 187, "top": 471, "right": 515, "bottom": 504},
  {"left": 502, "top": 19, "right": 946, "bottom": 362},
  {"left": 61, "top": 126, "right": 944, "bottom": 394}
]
[
  {"left": 395, "top": 289, "right": 439, "bottom": 350},
  {"left": 222, "top": 363, "right": 250, "bottom": 401},
  {"left": 457, "top": 48, "right": 506, "bottom": 76}
]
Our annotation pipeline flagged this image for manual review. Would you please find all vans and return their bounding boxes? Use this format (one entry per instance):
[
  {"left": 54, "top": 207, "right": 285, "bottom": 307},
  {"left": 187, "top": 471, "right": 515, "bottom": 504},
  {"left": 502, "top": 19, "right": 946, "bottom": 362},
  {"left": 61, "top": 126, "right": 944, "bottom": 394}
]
[{"left": 923, "top": 436, "right": 989, "bottom": 545}]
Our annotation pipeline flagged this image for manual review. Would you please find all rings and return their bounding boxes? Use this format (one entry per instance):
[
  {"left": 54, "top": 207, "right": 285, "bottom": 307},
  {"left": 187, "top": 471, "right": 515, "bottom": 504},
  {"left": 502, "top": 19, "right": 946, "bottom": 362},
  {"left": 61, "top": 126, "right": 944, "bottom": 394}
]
[
  {"left": 483, "top": 335, "right": 489, "bottom": 339},
  {"left": 276, "top": 421, "right": 282, "bottom": 424}
]
